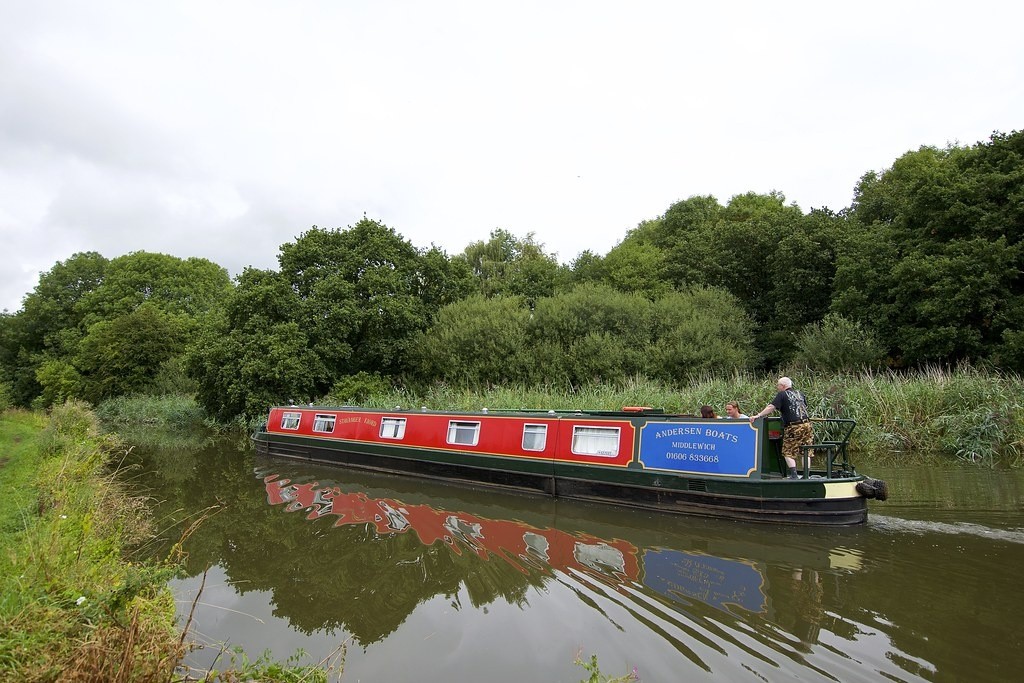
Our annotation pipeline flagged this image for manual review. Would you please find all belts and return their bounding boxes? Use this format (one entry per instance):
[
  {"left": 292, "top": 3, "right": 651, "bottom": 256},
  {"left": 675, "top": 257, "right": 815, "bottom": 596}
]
[{"left": 784, "top": 420, "right": 809, "bottom": 425}]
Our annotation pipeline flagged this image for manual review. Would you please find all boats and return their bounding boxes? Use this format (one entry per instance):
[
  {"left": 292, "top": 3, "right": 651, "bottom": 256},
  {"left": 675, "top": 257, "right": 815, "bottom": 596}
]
[{"left": 251, "top": 404, "right": 889, "bottom": 527}]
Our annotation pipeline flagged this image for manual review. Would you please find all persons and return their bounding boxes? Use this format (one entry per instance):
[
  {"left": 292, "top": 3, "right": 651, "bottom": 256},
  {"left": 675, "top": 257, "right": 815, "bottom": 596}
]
[
  {"left": 749, "top": 377, "right": 815, "bottom": 480},
  {"left": 701, "top": 406, "right": 723, "bottom": 419},
  {"left": 725, "top": 401, "right": 749, "bottom": 419}
]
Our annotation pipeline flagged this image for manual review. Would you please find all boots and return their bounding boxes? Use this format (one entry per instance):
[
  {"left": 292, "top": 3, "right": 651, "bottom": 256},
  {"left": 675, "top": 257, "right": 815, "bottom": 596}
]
[
  {"left": 800, "top": 468, "right": 809, "bottom": 479},
  {"left": 787, "top": 467, "right": 799, "bottom": 480}
]
